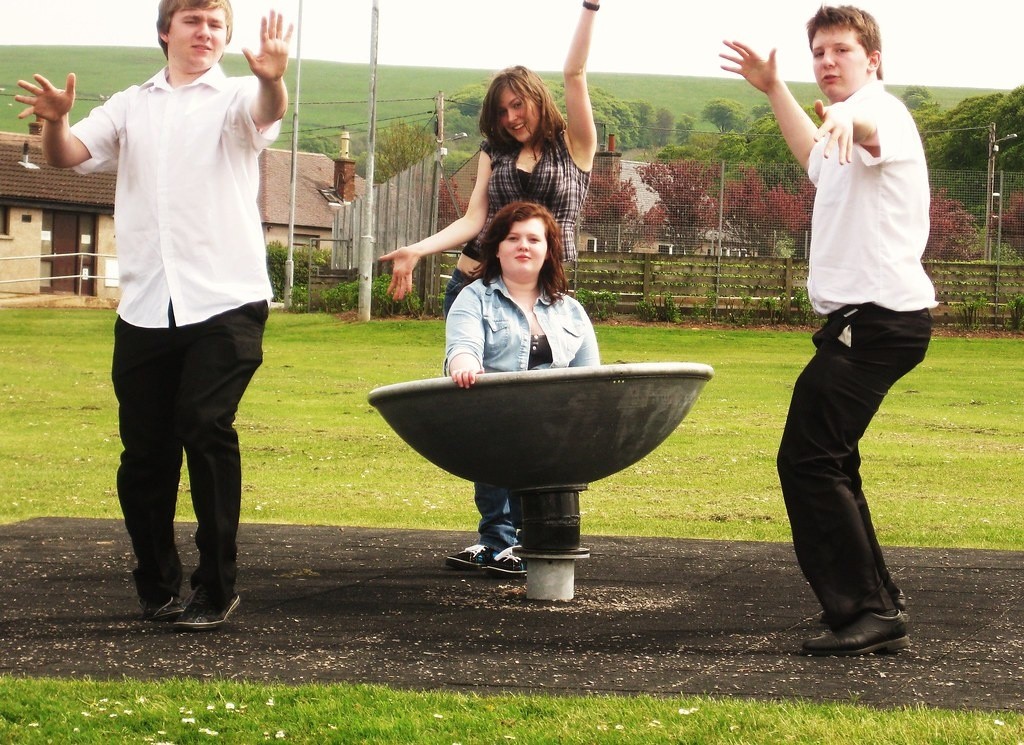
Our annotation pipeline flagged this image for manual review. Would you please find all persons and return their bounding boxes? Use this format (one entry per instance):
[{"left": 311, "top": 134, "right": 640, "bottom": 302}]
[
  {"left": 719, "top": 0, "right": 948, "bottom": 656},
  {"left": 441, "top": 194, "right": 606, "bottom": 390},
  {"left": 372, "top": 0, "right": 605, "bottom": 582},
  {"left": 14, "top": 0, "right": 299, "bottom": 635}
]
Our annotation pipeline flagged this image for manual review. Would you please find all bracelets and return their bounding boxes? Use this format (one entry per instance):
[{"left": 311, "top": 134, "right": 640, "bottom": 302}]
[{"left": 582, "top": 0, "right": 605, "bottom": 16}]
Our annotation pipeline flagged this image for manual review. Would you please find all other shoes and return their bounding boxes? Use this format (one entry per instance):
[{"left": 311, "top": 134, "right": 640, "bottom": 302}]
[
  {"left": 174, "top": 585, "right": 241, "bottom": 629},
  {"left": 445, "top": 545, "right": 502, "bottom": 567},
  {"left": 138, "top": 594, "right": 187, "bottom": 619},
  {"left": 486, "top": 545, "right": 527, "bottom": 573}
]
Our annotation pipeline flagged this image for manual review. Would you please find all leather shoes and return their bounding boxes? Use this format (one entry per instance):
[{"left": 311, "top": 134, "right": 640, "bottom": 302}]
[{"left": 802, "top": 588, "right": 909, "bottom": 659}]
